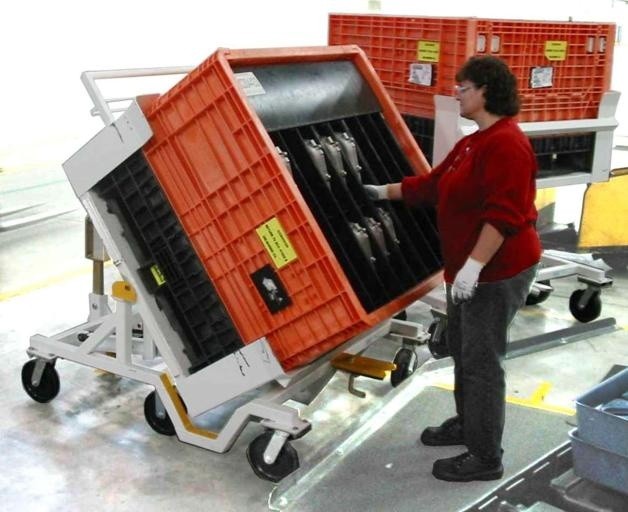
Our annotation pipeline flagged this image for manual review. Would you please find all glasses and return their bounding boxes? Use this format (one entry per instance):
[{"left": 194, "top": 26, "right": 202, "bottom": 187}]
[{"left": 453, "top": 85, "right": 473, "bottom": 94}]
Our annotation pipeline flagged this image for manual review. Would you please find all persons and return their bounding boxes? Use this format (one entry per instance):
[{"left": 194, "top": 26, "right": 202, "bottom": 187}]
[{"left": 363, "top": 46, "right": 541, "bottom": 481}]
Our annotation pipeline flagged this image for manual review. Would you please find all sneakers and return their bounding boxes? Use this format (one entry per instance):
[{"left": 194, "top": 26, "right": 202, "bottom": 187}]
[
  {"left": 421, "top": 415, "right": 467, "bottom": 446},
  {"left": 432, "top": 450, "right": 503, "bottom": 481}
]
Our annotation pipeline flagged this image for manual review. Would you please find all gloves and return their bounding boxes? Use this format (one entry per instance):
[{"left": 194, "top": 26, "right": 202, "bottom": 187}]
[
  {"left": 365, "top": 183, "right": 390, "bottom": 201},
  {"left": 451, "top": 257, "right": 484, "bottom": 305}
]
